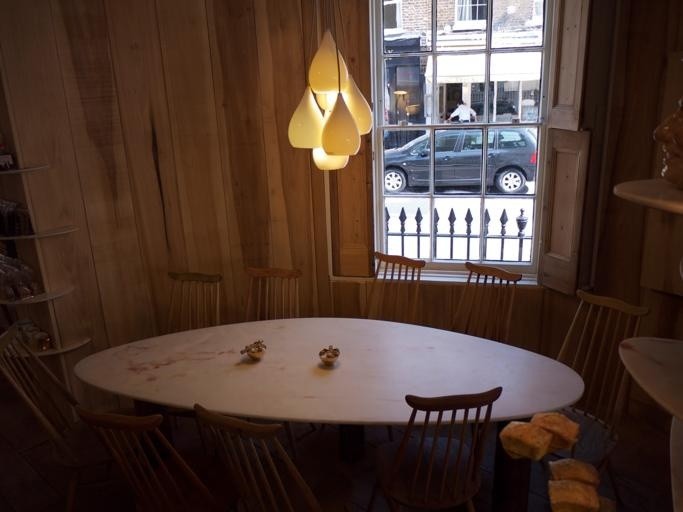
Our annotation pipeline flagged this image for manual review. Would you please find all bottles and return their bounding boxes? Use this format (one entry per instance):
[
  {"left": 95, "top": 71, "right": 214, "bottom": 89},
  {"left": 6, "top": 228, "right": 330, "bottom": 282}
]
[{"left": 16, "top": 320, "right": 49, "bottom": 351}]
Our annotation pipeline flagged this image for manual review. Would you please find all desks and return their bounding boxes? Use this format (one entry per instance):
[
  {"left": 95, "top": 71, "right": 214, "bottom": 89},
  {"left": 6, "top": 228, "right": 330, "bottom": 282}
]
[
  {"left": 610, "top": 174, "right": 681, "bottom": 214},
  {"left": 615, "top": 335, "right": 681, "bottom": 511},
  {"left": 70, "top": 313, "right": 588, "bottom": 428}
]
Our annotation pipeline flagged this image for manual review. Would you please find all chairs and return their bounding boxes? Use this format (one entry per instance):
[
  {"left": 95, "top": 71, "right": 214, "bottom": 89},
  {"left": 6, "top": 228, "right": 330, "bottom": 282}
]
[
  {"left": 544, "top": 288, "right": 649, "bottom": 510},
  {"left": 243, "top": 266, "right": 303, "bottom": 321},
  {"left": 163, "top": 271, "right": 223, "bottom": 332},
  {"left": 368, "top": 382, "right": 508, "bottom": 512},
  {"left": 445, "top": 261, "right": 521, "bottom": 345},
  {"left": 0, "top": 319, "right": 148, "bottom": 511},
  {"left": 71, "top": 404, "right": 217, "bottom": 510},
  {"left": 192, "top": 404, "right": 347, "bottom": 512},
  {"left": 367, "top": 248, "right": 425, "bottom": 323}
]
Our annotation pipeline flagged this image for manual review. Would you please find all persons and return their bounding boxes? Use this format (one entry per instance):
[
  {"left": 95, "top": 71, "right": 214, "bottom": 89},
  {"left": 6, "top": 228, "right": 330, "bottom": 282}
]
[{"left": 447, "top": 100, "right": 476, "bottom": 123}]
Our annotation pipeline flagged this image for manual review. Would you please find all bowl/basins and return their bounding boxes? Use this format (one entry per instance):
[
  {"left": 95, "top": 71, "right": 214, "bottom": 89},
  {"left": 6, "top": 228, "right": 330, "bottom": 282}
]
[
  {"left": 245, "top": 344, "right": 265, "bottom": 360},
  {"left": 318, "top": 348, "right": 339, "bottom": 366}
]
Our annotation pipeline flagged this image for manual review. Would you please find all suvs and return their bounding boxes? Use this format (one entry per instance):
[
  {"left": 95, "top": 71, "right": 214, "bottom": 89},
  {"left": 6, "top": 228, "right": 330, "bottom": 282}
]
[
  {"left": 472, "top": 99, "right": 516, "bottom": 115},
  {"left": 384, "top": 126, "right": 539, "bottom": 195}
]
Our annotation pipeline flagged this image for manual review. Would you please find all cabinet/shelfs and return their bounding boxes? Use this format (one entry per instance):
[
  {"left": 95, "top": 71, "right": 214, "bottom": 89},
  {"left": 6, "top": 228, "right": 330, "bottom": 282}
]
[{"left": 0, "top": 162, "right": 112, "bottom": 429}]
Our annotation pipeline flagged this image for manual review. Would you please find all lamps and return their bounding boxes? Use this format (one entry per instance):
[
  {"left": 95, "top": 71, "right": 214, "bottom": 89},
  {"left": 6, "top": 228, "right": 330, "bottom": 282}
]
[{"left": 286, "top": 0, "right": 375, "bottom": 172}]
